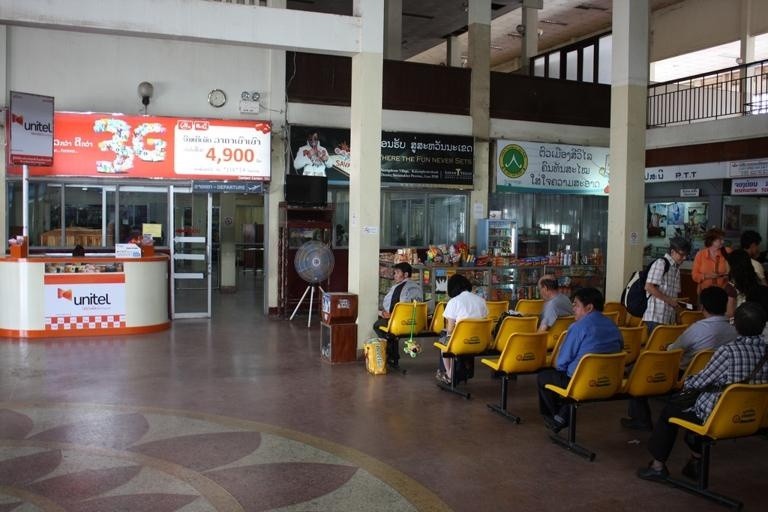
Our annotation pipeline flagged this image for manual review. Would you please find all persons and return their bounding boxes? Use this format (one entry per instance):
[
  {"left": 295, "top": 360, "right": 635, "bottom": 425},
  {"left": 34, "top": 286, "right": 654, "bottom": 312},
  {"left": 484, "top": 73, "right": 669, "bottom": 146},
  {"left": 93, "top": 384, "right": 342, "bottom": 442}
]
[
  {"left": 725, "top": 279, "right": 746, "bottom": 317},
  {"left": 293, "top": 129, "right": 333, "bottom": 178},
  {"left": 537, "top": 274, "right": 576, "bottom": 333},
  {"left": 437, "top": 274, "right": 490, "bottom": 385},
  {"left": 650, "top": 206, "right": 658, "bottom": 227},
  {"left": 636, "top": 302, "right": 767, "bottom": 486},
  {"left": 537, "top": 288, "right": 623, "bottom": 433},
  {"left": 721, "top": 230, "right": 767, "bottom": 321},
  {"left": 692, "top": 209, "right": 697, "bottom": 225},
  {"left": 641, "top": 238, "right": 691, "bottom": 336},
  {"left": 620, "top": 285, "right": 740, "bottom": 431},
  {"left": 692, "top": 228, "right": 734, "bottom": 295},
  {"left": 726, "top": 205, "right": 738, "bottom": 230},
  {"left": 373, "top": 262, "right": 424, "bottom": 364}
]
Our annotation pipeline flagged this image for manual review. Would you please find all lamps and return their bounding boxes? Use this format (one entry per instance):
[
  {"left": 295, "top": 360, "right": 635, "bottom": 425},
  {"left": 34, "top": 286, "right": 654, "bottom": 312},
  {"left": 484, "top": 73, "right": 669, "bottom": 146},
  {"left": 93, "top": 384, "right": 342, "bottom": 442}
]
[
  {"left": 240, "top": 92, "right": 260, "bottom": 101},
  {"left": 136, "top": 81, "right": 154, "bottom": 117}
]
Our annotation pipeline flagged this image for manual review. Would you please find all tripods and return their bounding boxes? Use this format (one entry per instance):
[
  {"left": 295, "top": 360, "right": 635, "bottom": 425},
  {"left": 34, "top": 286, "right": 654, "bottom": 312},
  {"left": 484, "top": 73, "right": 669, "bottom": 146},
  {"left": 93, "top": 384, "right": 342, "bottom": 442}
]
[{"left": 289, "top": 282, "right": 325, "bottom": 329}]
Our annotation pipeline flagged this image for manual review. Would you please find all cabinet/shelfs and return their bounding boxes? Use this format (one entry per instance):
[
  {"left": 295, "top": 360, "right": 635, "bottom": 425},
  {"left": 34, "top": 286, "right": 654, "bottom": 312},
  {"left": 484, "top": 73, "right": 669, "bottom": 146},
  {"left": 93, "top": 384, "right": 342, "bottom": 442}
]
[
  {"left": 491, "top": 265, "right": 542, "bottom": 303},
  {"left": 279, "top": 201, "right": 335, "bottom": 315},
  {"left": 543, "top": 265, "right": 606, "bottom": 297},
  {"left": 429, "top": 266, "right": 492, "bottom": 303},
  {"left": 378, "top": 259, "right": 433, "bottom": 314}
]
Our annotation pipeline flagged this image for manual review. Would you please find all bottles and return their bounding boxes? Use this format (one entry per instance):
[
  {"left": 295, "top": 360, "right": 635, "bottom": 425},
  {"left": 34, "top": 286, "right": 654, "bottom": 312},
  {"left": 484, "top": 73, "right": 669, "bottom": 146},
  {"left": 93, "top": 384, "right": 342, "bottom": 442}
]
[{"left": 550, "top": 244, "right": 601, "bottom": 265}]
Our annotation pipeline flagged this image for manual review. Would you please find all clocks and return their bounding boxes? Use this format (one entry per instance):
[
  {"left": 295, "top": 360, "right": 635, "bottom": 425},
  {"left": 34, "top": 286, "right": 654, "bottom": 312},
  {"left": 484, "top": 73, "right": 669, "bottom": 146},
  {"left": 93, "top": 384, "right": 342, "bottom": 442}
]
[{"left": 207, "top": 89, "right": 227, "bottom": 108}]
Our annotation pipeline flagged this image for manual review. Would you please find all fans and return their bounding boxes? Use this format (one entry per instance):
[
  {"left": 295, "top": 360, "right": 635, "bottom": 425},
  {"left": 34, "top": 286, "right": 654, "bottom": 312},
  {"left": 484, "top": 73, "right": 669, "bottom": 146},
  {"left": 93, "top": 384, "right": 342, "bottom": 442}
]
[{"left": 288, "top": 239, "right": 336, "bottom": 330}]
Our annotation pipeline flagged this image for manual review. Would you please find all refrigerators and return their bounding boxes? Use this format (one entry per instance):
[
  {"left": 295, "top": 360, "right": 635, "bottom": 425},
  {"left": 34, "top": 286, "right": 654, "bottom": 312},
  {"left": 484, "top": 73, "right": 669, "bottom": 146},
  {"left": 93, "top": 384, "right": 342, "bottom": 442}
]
[{"left": 475, "top": 218, "right": 517, "bottom": 260}]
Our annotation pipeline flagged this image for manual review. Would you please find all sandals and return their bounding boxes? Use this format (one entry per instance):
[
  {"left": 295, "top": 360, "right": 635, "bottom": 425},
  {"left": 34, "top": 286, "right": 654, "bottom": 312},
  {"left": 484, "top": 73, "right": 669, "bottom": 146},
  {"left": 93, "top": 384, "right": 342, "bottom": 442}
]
[{"left": 441, "top": 374, "right": 452, "bottom": 385}]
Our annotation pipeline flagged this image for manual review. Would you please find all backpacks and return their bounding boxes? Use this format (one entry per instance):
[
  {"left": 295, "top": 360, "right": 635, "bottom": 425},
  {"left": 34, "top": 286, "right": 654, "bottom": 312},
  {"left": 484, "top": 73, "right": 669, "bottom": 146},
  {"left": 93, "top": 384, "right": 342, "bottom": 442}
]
[{"left": 620, "top": 256, "right": 671, "bottom": 318}]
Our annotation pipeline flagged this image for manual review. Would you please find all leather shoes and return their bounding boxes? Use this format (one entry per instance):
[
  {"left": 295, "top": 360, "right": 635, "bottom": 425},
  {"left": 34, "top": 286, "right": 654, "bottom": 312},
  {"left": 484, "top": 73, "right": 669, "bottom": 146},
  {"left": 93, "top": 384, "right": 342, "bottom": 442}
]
[
  {"left": 637, "top": 452, "right": 711, "bottom": 489},
  {"left": 544, "top": 415, "right": 569, "bottom": 436},
  {"left": 621, "top": 417, "right": 653, "bottom": 432}
]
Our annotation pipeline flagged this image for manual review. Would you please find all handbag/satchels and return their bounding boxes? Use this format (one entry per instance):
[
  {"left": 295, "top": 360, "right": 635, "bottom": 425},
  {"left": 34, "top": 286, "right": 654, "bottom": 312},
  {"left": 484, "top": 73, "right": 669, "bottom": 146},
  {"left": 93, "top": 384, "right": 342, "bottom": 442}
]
[
  {"left": 669, "top": 388, "right": 703, "bottom": 410},
  {"left": 492, "top": 312, "right": 523, "bottom": 341},
  {"left": 426, "top": 298, "right": 435, "bottom": 316}
]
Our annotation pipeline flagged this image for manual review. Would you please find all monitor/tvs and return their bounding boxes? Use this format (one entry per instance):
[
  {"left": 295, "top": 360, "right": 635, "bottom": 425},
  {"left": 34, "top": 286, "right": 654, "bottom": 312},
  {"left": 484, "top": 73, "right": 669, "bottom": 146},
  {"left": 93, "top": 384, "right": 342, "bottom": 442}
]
[{"left": 283, "top": 174, "right": 328, "bottom": 209}]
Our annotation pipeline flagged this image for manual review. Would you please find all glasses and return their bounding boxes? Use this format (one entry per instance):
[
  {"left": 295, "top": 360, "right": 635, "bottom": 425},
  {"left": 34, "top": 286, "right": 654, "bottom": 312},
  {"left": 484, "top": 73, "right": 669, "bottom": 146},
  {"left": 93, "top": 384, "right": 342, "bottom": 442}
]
[{"left": 674, "top": 249, "right": 685, "bottom": 258}]
